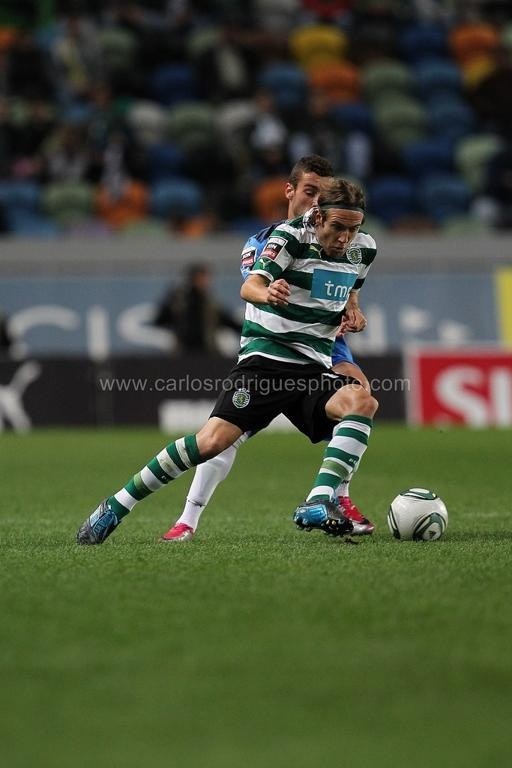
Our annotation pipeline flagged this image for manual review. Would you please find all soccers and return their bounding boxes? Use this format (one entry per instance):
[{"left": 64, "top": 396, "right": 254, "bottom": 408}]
[{"left": 388, "top": 488, "right": 447, "bottom": 541}]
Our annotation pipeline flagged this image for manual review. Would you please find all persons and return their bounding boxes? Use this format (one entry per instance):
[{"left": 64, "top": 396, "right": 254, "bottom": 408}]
[
  {"left": 0, "top": 1, "right": 511, "bottom": 238},
  {"left": 152, "top": 264, "right": 244, "bottom": 396},
  {"left": 156, "top": 154, "right": 375, "bottom": 542},
  {"left": 75, "top": 178, "right": 380, "bottom": 546}
]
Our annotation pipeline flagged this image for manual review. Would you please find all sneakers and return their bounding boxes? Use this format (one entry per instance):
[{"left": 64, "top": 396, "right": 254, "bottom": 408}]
[
  {"left": 293, "top": 499, "right": 353, "bottom": 537},
  {"left": 78, "top": 498, "right": 122, "bottom": 546},
  {"left": 336, "top": 495, "right": 375, "bottom": 535},
  {"left": 159, "top": 524, "right": 195, "bottom": 544}
]
